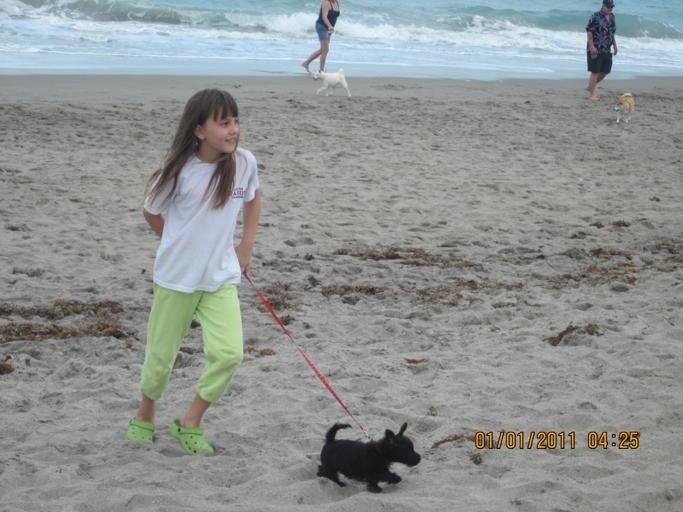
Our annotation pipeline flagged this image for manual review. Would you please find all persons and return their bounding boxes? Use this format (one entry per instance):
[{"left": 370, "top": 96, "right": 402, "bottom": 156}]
[
  {"left": 302, "top": 0, "right": 340, "bottom": 72},
  {"left": 127, "top": 90, "right": 262, "bottom": 456},
  {"left": 585, "top": 0, "right": 617, "bottom": 101}
]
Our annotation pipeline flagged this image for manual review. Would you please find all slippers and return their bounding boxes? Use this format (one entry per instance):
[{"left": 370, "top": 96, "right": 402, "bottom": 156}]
[
  {"left": 168, "top": 419, "right": 215, "bottom": 456},
  {"left": 125, "top": 416, "right": 155, "bottom": 442}
]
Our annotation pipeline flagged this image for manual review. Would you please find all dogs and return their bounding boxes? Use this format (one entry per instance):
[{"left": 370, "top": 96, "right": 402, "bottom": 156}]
[
  {"left": 312, "top": 68, "right": 352, "bottom": 100},
  {"left": 612, "top": 92, "right": 635, "bottom": 125},
  {"left": 316, "top": 421, "right": 422, "bottom": 493}
]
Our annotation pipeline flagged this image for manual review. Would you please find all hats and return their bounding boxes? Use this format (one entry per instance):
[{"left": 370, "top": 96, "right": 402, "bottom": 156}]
[{"left": 603, "top": 0, "right": 615, "bottom": 7}]
